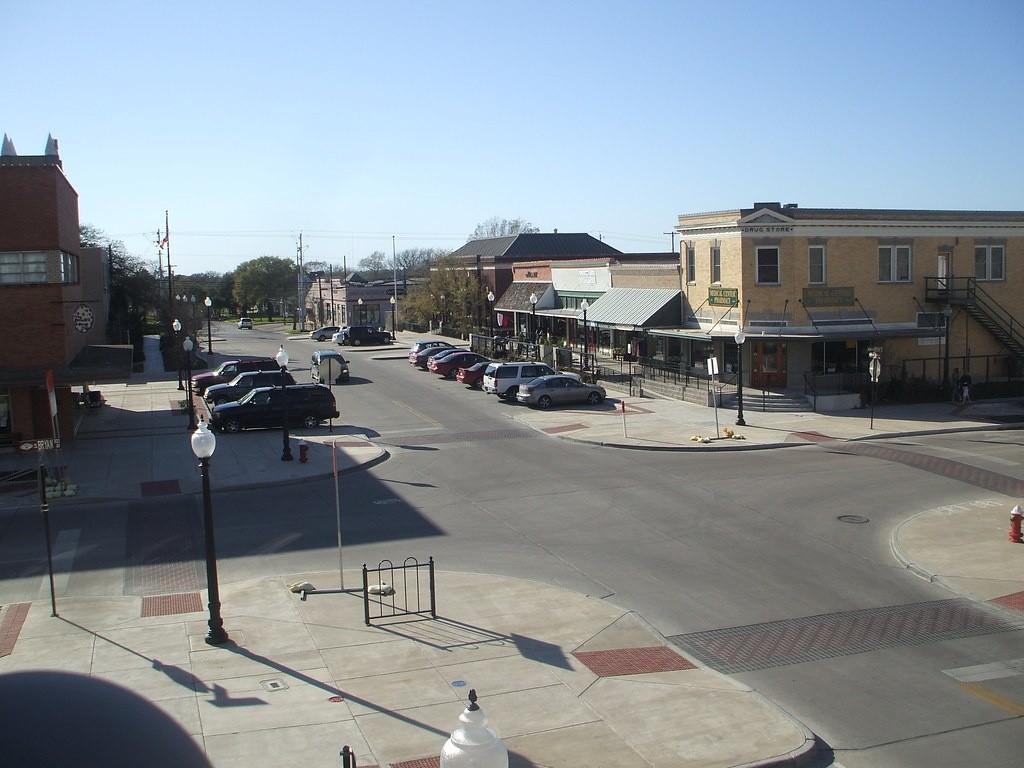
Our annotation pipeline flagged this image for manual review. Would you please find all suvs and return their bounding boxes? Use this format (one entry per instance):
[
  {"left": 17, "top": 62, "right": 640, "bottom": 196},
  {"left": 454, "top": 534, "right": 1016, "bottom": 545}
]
[{"left": 481, "top": 360, "right": 562, "bottom": 402}]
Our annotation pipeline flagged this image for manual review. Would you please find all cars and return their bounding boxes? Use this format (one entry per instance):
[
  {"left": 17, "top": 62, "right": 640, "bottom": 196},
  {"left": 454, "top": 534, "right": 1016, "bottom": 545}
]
[
  {"left": 237, "top": 318, "right": 253, "bottom": 330},
  {"left": 409, "top": 340, "right": 501, "bottom": 390},
  {"left": 194, "top": 325, "right": 391, "bottom": 434},
  {"left": 516, "top": 374, "right": 606, "bottom": 410}
]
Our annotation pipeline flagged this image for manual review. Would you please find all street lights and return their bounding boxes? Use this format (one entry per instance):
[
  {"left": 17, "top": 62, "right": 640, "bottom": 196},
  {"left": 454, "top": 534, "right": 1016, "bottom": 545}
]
[
  {"left": 358, "top": 297, "right": 362, "bottom": 326},
  {"left": 190, "top": 295, "right": 195, "bottom": 341},
  {"left": 183, "top": 336, "right": 197, "bottom": 430},
  {"left": 530, "top": 293, "right": 538, "bottom": 342},
  {"left": 390, "top": 296, "right": 396, "bottom": 340},
  {"left": 735, "top": 325, "right": 746, "bottom": 425},
  {"left": 487, "top": 291, "right": 495, "bottom": 336},
  {"left": 191, "top": 415, "right": 229, "bottom": 645},
  {"left": 942, "top": 304, "right": 952, "bottom": 399},
  {"left": 275, "top": 345, "right": 293, "bottom": 460},
  {"left": 581, "top": 299, "right": 590, "bottom": 371},
  {"left": 173, "top": 318, "right": 184, "bottom": 390},
  {"left": 205, "top": 296, "right": 212, "bottom": 354}
]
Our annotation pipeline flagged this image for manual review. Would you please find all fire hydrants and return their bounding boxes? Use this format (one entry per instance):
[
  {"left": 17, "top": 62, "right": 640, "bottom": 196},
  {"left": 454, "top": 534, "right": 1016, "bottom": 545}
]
[
  {"left": 1009, "top": 504, "right": 1024, "bottom": 543},
  {"left": 298, "top": 437, "right": 308, "bottom": 462}
]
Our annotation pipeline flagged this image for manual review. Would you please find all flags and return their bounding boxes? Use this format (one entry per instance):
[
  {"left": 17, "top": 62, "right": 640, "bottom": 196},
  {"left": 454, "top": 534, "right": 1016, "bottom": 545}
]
[{"left": 497, "top": 312, "right": 507, "bottom": 327}]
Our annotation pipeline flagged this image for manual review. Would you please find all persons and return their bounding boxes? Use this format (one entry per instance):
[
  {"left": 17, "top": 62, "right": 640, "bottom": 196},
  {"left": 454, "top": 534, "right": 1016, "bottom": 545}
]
[
  {"left": 890, "top": 367, "right": 974, "bottom": 406},
  {"left": 493, "top": 327, "right": 546, "bottom": 346}
]
